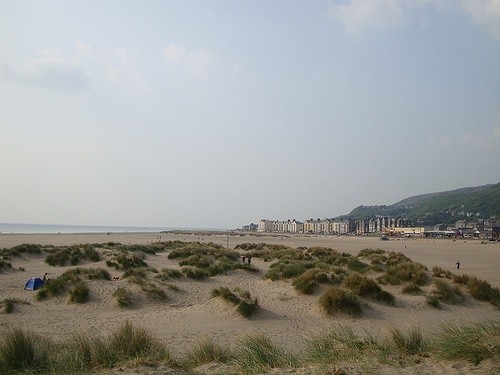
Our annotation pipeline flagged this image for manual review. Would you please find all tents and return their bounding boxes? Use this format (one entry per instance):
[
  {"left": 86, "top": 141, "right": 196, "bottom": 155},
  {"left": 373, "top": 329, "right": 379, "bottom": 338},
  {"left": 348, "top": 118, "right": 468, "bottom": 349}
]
[{"left": 23, "top": 277, "right": 46, "bottom": 292}]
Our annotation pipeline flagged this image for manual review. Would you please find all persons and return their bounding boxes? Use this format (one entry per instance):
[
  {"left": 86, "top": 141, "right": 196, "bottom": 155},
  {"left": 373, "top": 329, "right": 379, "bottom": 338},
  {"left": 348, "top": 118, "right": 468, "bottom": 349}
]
[{"left": 43, "top": 273, "right": 48, "bottom": 281}]
